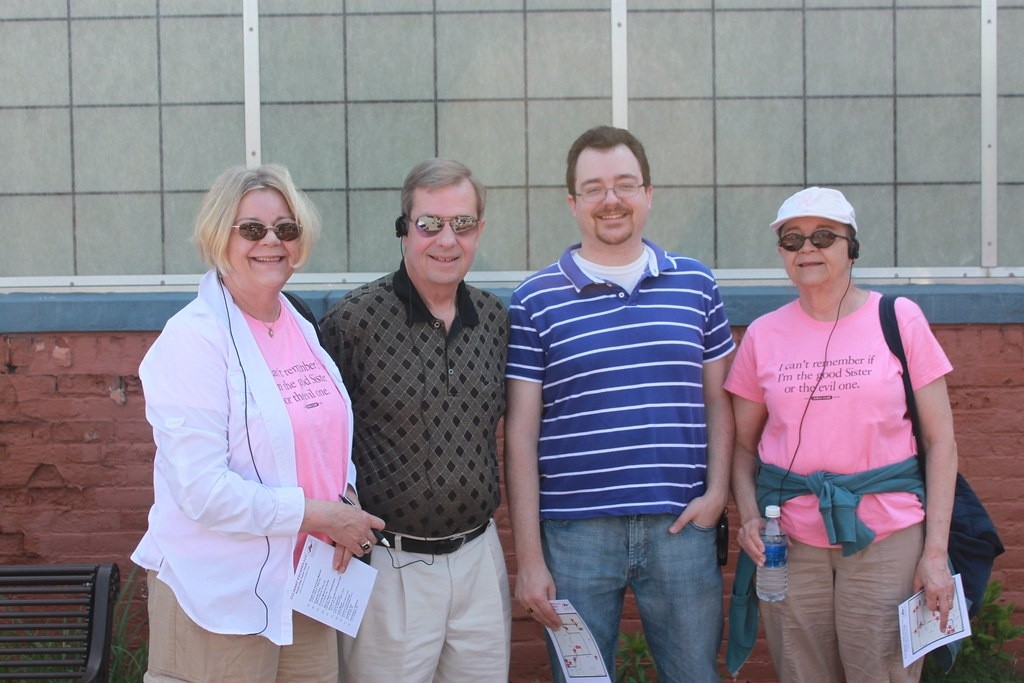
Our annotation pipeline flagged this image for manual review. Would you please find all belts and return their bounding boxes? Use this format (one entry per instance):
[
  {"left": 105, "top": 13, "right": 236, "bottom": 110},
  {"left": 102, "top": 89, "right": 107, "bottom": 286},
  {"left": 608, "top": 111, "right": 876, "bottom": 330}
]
[{"left": 368, "top": 517, "right": 490, "bottom": 556}]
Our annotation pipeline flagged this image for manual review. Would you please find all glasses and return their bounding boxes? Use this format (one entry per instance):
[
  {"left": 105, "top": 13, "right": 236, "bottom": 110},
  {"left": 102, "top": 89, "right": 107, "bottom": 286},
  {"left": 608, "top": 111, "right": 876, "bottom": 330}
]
[
  {"left": 777, "top": 229, "right": 853, "bottom": 252},
  {"left": 575, "top": 183, "right": 646, "bottom": 203},
  {"left": 232, "top": 221, "right": 302, "bottom": 241},
  {"left": 408, "top": 215, "right": 481, "bottom": 233}
]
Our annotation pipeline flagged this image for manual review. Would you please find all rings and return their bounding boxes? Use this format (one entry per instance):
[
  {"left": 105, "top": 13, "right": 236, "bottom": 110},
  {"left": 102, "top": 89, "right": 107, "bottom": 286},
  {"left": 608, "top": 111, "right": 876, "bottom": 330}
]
[
  {"left": 948, "top": 600, "right": 952, "bottom": 602},
  {"left": 526, "top": 608, "right": 533, "bottom": 614},
  {"left": 362, "top": 541, "right": 370, "bottom": 549}
]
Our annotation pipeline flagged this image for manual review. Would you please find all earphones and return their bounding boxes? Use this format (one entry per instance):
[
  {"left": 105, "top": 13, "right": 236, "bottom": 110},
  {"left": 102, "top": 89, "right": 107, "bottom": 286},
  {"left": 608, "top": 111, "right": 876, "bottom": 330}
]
[
  {"left": 847, "top": 244, "right": 859, "bottom": 260},
  {"left": 395, "top": 215, "right": 408, "bottom": 238}
]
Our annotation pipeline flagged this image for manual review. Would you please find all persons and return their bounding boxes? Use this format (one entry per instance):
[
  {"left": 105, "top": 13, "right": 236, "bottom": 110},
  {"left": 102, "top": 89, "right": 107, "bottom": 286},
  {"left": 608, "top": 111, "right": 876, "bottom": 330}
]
[
  {"left": 129, "top": 165, "right": 386, "bottom": 683},
  {"left": 319, "top": 158, "right": 511, "bottom": 682},
  {"left": 723, "top": 187, "right": 958, "bottom": 683},
  {"left": 504, "top": 127, "right": 733, "bottom": 683}
]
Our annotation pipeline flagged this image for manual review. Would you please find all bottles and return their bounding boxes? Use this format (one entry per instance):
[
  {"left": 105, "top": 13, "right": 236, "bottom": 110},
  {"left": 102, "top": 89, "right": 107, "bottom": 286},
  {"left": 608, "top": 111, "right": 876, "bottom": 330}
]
[{"left": 754, "top": 504, "right": 789, "bottom": 602}]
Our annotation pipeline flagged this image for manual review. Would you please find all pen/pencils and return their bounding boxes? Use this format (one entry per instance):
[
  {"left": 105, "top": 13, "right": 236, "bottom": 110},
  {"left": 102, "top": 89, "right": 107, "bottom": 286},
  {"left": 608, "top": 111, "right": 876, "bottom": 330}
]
[{"left": 339, "top": 494, "right": 391, "bottom": 547}]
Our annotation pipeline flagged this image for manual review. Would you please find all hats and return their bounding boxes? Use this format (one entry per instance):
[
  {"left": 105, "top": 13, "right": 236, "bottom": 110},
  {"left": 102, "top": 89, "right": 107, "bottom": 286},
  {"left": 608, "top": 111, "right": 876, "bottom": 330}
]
[{"left": 770, "top": 185, "right": 857, "bottom": 238}]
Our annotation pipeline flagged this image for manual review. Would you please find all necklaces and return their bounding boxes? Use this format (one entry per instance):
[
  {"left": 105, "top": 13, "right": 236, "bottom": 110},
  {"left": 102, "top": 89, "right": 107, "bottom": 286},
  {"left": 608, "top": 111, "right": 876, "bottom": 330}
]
[{"left": 243, "top": 303, "right": 278, "bottom": 337}]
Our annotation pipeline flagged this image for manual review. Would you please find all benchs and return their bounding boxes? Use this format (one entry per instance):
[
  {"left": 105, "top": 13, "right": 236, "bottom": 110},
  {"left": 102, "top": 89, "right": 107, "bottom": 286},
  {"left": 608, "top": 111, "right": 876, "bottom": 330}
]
[{"left": 0, "top": 557, "right": 124, "bottom": 683}]
